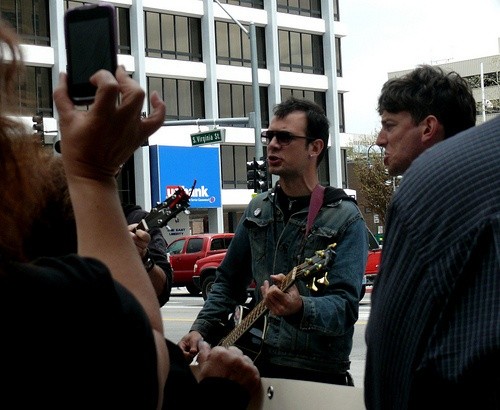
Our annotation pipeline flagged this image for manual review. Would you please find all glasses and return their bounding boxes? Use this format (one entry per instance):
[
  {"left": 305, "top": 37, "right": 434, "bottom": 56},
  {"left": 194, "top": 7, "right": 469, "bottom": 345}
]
[{"left": 260, "top": 130, "right": 317, "bottom": 146}]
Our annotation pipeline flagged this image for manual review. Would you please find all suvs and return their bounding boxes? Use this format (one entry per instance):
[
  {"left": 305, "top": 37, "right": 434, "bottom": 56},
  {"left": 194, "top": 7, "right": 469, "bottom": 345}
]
[{"left": 166, "top": 232, "right": 258, "bottom": 302}]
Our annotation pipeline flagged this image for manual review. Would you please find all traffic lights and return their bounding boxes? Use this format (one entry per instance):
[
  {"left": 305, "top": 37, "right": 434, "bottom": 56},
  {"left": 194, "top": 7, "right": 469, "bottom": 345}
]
[
  {"left": 255, "top": 156, "right": 270, "bottom": 193},
  {"left": 32, "top": 112, "right": 45, "bottom": 146},
  {"left": 245, "top": 156, "right": 258, "bottom": 194}
]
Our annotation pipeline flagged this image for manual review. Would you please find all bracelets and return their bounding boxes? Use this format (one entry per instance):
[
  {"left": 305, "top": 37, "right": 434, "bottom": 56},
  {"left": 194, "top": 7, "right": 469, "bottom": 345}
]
[{"left": 143, "top": 250, "right": 154, "bottom": 272}]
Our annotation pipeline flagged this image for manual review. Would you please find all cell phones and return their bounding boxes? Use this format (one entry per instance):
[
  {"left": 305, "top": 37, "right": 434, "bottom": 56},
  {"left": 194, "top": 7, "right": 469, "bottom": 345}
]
[{"left": 63, "top": 2, "right": 118, "bottom": 106}]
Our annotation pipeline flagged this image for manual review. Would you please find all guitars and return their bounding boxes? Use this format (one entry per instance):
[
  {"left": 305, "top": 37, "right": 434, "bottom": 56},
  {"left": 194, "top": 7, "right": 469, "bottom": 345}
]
[
  {"left": 190, "top": 242, "right": 337, "bottom": 384},
  {"left": 130, "top": 187, "right": 190, "bottom": 235}
]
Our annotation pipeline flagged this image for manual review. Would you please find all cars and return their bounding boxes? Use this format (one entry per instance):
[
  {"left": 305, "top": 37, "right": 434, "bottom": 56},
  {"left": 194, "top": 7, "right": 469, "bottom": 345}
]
[{"left": 359, "top": 225, "right": 384, "bottom": 303}]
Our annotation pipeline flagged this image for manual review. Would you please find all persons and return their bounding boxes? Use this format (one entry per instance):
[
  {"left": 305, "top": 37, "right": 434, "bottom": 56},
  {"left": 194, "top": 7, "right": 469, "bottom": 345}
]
[
  {"left": 364, "top": 113, "right": 500, "bottom": 410},
  {"left": 0, "top": 26, "right": 170, "bottom": 410},
  {"left": 180, "top": 97, "right": 369, "bottom": 386},
  {"left": 165, "top": 336, "right": 263, "bottom": 410},
  {"left": 54, "top": 139, "right": 173, "bottom": 306},
  {"left": 375, "top": 66, "right": 476, "bottom": 176}
]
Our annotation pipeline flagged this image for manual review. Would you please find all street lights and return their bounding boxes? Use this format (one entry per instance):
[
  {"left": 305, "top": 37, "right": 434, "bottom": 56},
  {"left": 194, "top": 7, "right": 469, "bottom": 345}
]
[{"left": 480, "top": 95, "right": 494, "bottom": 124}]
